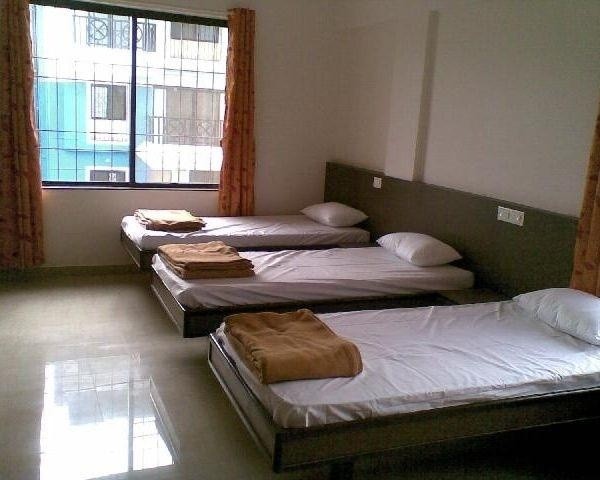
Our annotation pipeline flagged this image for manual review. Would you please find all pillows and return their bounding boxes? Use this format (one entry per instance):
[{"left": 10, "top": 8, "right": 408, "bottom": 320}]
[{"left": 300, "top": 202, "right": 371, "bottom": 228}]
